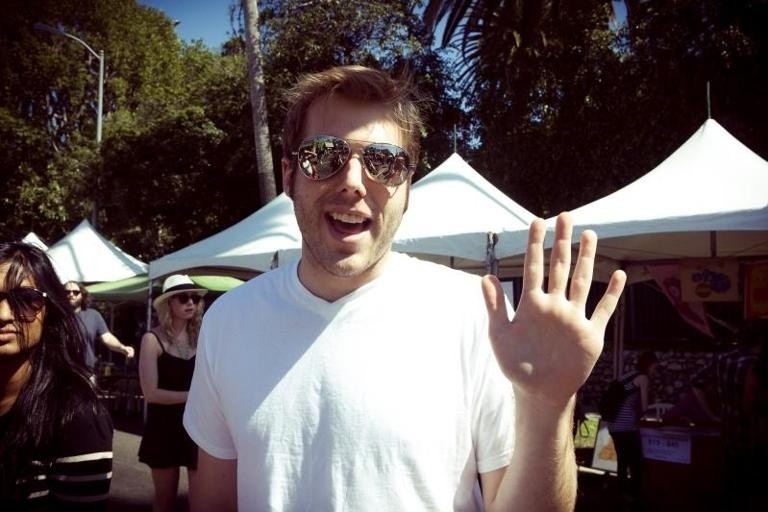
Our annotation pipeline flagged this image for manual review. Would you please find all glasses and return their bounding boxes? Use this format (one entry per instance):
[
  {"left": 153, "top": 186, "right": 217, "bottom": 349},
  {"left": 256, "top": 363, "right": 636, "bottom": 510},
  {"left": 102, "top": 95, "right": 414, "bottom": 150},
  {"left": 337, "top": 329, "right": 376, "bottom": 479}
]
[
  {"left": 7, "top": 288, "right": 47, "bottom": 314},
  {"left": 65, "top": 291, "right": 81, "bottom": 295},
  {"left": 292, "top": 135, "right": 415, "bottom": 187},
  {"left": 179, "top": 293, "right": 201, "bottom": 304}
]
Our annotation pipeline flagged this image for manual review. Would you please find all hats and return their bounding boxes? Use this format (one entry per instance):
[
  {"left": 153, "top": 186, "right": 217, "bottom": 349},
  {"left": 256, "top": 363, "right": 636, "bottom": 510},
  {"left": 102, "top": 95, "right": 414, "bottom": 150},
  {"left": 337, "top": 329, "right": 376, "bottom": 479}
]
[{"left": 153, "top": 274, "right": 208, "bottom": 311}]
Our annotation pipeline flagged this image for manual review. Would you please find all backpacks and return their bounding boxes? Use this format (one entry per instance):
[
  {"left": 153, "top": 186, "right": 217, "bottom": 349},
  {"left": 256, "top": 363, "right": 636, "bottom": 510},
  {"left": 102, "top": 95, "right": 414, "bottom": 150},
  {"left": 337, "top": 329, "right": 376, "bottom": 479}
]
[{"left": 600, "top": 371, "right": 641, "bottom": 421}]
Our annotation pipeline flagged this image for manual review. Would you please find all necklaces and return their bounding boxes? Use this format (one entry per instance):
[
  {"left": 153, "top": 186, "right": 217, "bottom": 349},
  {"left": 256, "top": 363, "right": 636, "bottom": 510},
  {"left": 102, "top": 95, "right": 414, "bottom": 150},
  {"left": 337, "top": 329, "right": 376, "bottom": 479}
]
[{"left": 174, "top": 338, "right": 191, "bottom": 360}]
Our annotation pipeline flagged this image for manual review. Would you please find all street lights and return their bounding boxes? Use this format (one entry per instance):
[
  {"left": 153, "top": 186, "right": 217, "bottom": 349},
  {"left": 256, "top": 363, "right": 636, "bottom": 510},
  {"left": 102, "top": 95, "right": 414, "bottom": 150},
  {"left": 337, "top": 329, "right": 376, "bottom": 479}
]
[{"left": 32, "top": 22, "right": 105, "bottom": 228}]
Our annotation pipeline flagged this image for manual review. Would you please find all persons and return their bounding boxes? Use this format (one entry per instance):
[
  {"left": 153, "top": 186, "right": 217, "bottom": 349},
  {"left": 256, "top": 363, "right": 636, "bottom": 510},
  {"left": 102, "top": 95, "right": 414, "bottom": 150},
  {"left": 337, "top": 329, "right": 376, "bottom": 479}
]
[
  {"left": 608, "top": 354, "right": 658, "bottom": 491},
  {"left": 179, "top": 61, "right": 629, "bottom": 512},
  {"left": 137, "top": 275, "right": 208, "bottom": 511},
  {"left": 0, "top": 243, "right": 114, "bottom": 512},
  {"left": 62, "top": 281, "right": 136, "bottom": 390}
]
